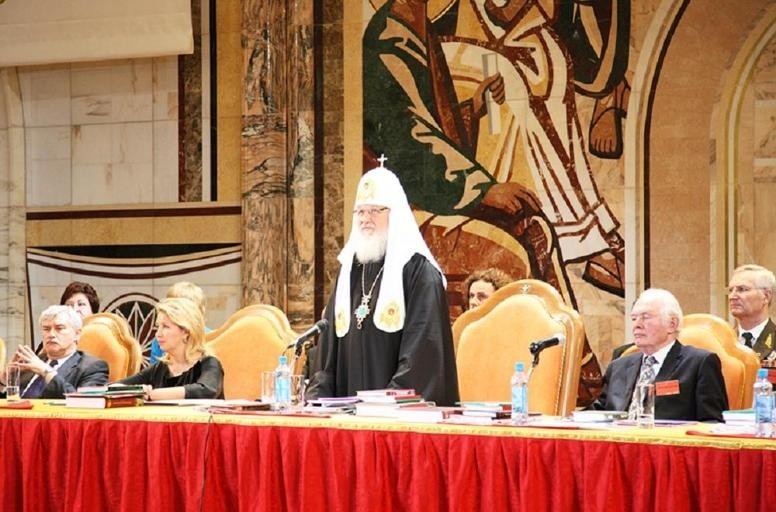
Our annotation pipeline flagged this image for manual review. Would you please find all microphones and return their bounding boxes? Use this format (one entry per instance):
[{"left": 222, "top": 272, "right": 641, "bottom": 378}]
[
  {"left": 287, "top": 319, "right": 329, "bottom": 349},
  {"left": 528, "top": 333, "right": 567, "bottom": 355}
]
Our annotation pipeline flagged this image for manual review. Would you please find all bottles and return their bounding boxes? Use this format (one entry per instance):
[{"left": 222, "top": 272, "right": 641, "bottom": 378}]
[
  {"left": 511, "top": 363, "right": 528, "bottom": 422},
  {"left": 275, "top": 358, "right": 290, "bottom": 406},
  {"left": 754, "top": 370, "right": 772, "bottom": 437}
]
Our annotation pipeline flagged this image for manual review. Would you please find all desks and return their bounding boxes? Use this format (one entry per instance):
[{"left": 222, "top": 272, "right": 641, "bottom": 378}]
[{"left": 0, "top": 391, "right": 773, "bottom": 512}]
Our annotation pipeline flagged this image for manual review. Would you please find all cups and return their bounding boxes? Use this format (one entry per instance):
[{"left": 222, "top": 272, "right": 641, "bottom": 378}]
[
  {"left": 634, "top": 383, "right": 657, "bottom": 430},
  {"left": 4, "top": 365, "right": 21, "bottom": 402},
  {"left": 289, "top": 372, "right": 303, "bottom": 405},
  {"left": 262, "top": 370, "right": 275, "bottom": 406}
]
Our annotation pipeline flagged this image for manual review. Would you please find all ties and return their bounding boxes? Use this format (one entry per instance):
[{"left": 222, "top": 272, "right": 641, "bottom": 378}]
[
  {"left": 628, "top": 356, "right": 656, "bottom": 414},
  {"left": 21, "top": 359, "right": 59, "bottom": 398},
  {"left": 742, "top": 332, "right": 753, "bottom": 350}
]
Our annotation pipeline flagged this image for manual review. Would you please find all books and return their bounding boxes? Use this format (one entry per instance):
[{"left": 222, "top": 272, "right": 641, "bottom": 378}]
[
  {"left": 450, "top": 400, "right": 541, "bottom": 421},
  {"left": 722, "top": 409, "right": 758, "bottom": 427},
  {"left": 64, "top": 385, "right": 155, "bottom": 409},
  {"left": 349, "top": 389, "right": 451, "bottom": 421}
]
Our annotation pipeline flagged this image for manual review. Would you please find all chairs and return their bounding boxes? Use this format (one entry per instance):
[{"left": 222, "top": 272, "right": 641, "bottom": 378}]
[
  {"left": 620, "top": 313, "right": 762, "bottom": 410},
  {"left": 451, "top": 279, "right": 586, "bottom": 418},
  {"left": 76, "top": 312, "right": 143, "bottom": 383},
  {"left": 204, "top": 304, "right": 307, "bottom": 401}
]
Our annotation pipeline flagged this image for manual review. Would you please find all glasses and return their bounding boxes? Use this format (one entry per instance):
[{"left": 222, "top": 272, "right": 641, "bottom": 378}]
[
  {"left": 355, "top": 206, "right": 389, "bottom": 217},
  {"left": 724, "top": 285, "right": 768, "bottom": 296}
]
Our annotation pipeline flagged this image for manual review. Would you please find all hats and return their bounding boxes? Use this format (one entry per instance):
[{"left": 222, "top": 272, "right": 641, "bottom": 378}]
[{"left": 333, "top": 166, "right": 447, "bottom": 338}]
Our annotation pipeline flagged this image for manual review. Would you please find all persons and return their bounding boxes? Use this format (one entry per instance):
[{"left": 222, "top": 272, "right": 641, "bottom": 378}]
[
  {"left": 306, "top": 165, "right": 459, "bottom": 406},
  {"left": 359, "top": 0, "right": 628, "bottom": 409},
  {"left": 552, "top": 0, "right": 633, "bottom": 160},
  {"left": 462, "top": 268, "right": 515, "bottom": 310},
  {"left": 150, "top": 281, "right": 216, "bottom": 365},
  {"left": 1, "top": 305, "right": 110, "bottom": 399},
  {"left": 579, "top": 287, "right": 730, "bottom": 422},
  {"left": 107, "top": 297, "right": 224, "bottom": 399},
  {"left": 427, "top": 0, "right": 626, "bottom": 299},
  {"left": 35, "top": 282, "right": 99, "bottom": 359},
  {"left": 727, "top": 264, "right": 776, "bottom": 368}
]
